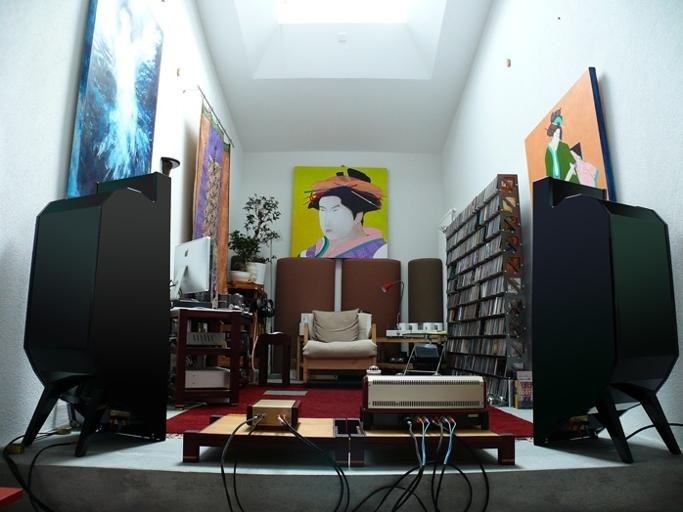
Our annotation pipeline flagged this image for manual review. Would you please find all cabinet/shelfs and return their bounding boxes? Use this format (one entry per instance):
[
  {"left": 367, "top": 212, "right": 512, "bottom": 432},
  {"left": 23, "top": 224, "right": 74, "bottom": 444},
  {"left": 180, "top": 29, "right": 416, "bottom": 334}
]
[
  {"left": 177, "top": 308, "right": 254, "bottom": 406},
  {"left": 444, "top": 174, "right": 526, "bottom": 404}
]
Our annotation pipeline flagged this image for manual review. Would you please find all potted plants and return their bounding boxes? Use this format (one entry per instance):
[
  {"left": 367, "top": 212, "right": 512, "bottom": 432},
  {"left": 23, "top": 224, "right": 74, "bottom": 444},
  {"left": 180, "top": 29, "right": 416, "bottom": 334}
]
[{"left": 227, "top": 193, "right": 283, "bottom": 287}]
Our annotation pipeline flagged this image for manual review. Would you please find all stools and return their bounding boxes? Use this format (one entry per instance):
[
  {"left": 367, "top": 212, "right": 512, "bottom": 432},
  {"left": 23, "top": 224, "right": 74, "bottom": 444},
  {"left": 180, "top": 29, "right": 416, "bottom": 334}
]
[{"left": 260, "top": 333, "right": 292, "bottom": 386}]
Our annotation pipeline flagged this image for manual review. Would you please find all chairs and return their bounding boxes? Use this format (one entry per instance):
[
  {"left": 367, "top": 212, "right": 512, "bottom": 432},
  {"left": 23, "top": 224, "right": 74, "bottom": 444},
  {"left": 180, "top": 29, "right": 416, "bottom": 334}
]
[{"left": 296, "top": 310, "right": 378, "bottom": 387}]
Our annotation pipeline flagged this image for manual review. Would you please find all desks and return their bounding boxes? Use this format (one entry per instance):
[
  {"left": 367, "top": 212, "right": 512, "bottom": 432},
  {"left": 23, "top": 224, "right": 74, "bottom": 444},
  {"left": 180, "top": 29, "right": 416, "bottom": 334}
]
[{"left": 377, "top": 337, "right": 447, "bottom": 376}]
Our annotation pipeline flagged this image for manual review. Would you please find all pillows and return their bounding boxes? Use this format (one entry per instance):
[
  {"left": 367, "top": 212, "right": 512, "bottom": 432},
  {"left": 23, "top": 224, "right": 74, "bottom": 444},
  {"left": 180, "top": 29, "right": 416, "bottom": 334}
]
[{"left": 312, "top": 307, "right": 362, "bottom": 342}]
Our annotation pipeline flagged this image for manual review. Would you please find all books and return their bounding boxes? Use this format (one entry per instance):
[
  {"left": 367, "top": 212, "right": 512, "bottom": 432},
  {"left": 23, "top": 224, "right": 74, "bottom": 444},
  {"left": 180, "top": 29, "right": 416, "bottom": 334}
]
[{"left": 442, "top": 173, "right": 533, "bottom": 411}]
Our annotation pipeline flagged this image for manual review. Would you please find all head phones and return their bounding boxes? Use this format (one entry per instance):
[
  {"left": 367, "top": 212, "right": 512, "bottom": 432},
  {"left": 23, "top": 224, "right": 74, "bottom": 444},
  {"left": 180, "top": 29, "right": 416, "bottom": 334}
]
[{"left": 259, "top": 299, "right": 275, "bottom": 318}]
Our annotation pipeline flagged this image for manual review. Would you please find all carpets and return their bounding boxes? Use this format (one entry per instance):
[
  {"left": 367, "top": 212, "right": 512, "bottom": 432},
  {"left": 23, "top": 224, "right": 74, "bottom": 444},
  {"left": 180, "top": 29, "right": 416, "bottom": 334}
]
[{"left": 166, "top": 382, "right": 535, "bottom": 439}]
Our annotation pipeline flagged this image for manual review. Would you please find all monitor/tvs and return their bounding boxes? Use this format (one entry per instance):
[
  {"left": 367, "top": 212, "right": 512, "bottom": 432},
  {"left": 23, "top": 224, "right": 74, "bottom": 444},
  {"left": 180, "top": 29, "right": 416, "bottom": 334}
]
[
  {"left": 20, "top": 172, "right": 171, "bottom": 458},
  {"left": 532, "top": 176, "right": 681, "bottom": 464},
  {"left": 170, "top": 236, "right": 211, "bottom": 302}
]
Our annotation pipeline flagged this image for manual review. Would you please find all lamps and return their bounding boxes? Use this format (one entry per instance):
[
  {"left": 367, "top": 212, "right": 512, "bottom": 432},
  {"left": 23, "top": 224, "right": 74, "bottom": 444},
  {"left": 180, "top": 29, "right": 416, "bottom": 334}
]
[{"left": 380, "top": 280, "right": 405, "bottom": 330}]
